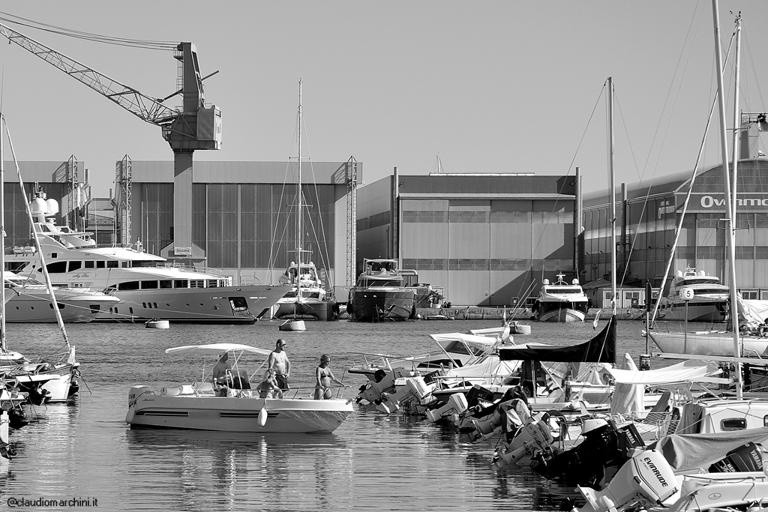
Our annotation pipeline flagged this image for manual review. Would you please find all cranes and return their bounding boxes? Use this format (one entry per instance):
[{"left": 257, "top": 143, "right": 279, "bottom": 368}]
[{"left": 0, "top": 11, "right": 222, "bottom": 258}]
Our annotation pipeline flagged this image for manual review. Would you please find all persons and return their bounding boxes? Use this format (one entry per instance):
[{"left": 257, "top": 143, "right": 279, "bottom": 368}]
[
  {"left": 256, "top": 339, "right": 291, "bottom": 398},
  {"left": 314, "top": 354, "right": 345, "bottom": 399},
  {"left": 212, "top": 351, "right": 231, "bottom": 397}
]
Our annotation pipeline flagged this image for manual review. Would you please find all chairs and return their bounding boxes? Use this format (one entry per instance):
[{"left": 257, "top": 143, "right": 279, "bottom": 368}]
[{"left": 226, "top": 369, "right": 251, "bottom": 397}]
